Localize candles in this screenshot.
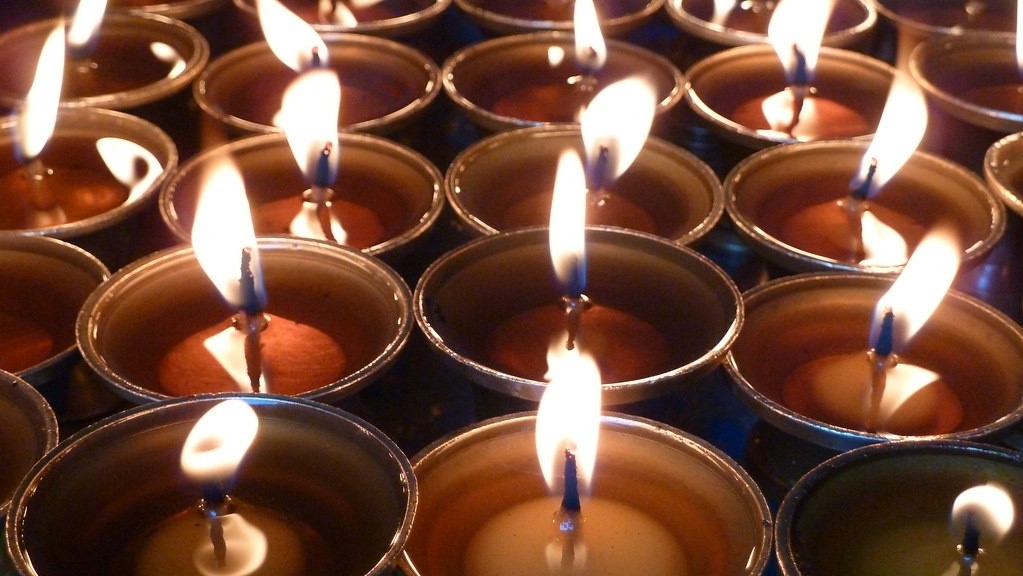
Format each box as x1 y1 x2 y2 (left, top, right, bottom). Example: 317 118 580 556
0 0 1023 576
